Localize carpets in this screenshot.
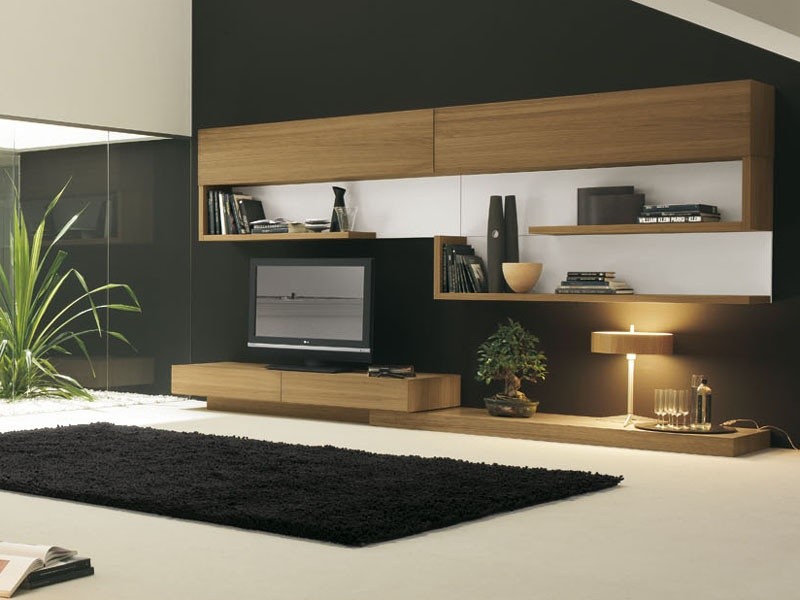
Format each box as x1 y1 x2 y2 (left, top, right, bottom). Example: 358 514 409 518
0 422 626 549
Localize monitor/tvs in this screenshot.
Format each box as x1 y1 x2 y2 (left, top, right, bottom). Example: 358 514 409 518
248 257 374 373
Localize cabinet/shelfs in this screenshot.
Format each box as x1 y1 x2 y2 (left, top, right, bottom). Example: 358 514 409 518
197 78 775 308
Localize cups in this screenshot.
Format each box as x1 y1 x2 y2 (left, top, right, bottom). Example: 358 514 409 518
334 206 358 232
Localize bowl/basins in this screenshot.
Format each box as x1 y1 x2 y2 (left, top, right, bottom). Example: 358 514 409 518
501 262 543 293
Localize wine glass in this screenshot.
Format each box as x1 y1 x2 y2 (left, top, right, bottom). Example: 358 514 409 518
652 374 705 431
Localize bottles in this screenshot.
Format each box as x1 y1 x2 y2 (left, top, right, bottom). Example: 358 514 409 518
330 186 349 233
694 378 713 431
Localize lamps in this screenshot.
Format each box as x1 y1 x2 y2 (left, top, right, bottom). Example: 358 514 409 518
590 326 676 427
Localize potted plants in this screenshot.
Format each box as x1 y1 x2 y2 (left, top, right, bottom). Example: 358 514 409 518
473 319 548 417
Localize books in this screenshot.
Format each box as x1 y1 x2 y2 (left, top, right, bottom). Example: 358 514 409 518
0 542 95 598
555 272 635 294
208 191 267 235
442 243 487 293
638 203 721 222
249 218 290 233
